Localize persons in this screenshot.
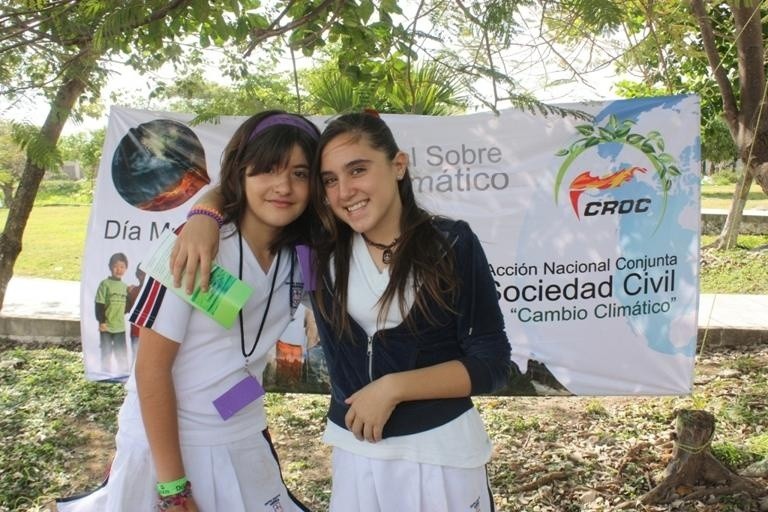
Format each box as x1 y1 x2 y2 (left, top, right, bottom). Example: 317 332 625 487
168 106 512 512
125 261 147 368
274 303 316 392
55 109 322 512
95 252 128 372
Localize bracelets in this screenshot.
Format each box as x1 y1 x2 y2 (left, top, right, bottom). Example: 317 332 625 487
188 203 225 228
154 478 192 511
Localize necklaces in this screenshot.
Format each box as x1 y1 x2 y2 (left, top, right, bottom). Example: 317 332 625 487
360 231 398 264
